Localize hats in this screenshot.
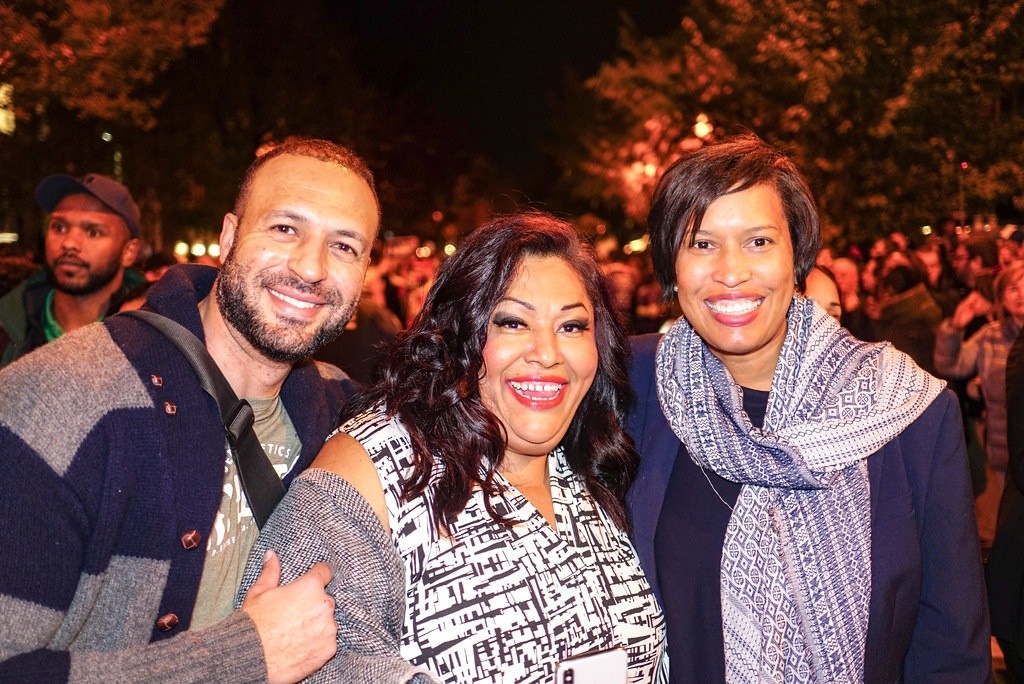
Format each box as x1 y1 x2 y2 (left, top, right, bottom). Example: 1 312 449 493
35 173 141 238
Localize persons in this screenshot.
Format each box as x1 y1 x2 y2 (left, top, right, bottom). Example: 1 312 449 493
0 136 1024 684
232 210 673 684
